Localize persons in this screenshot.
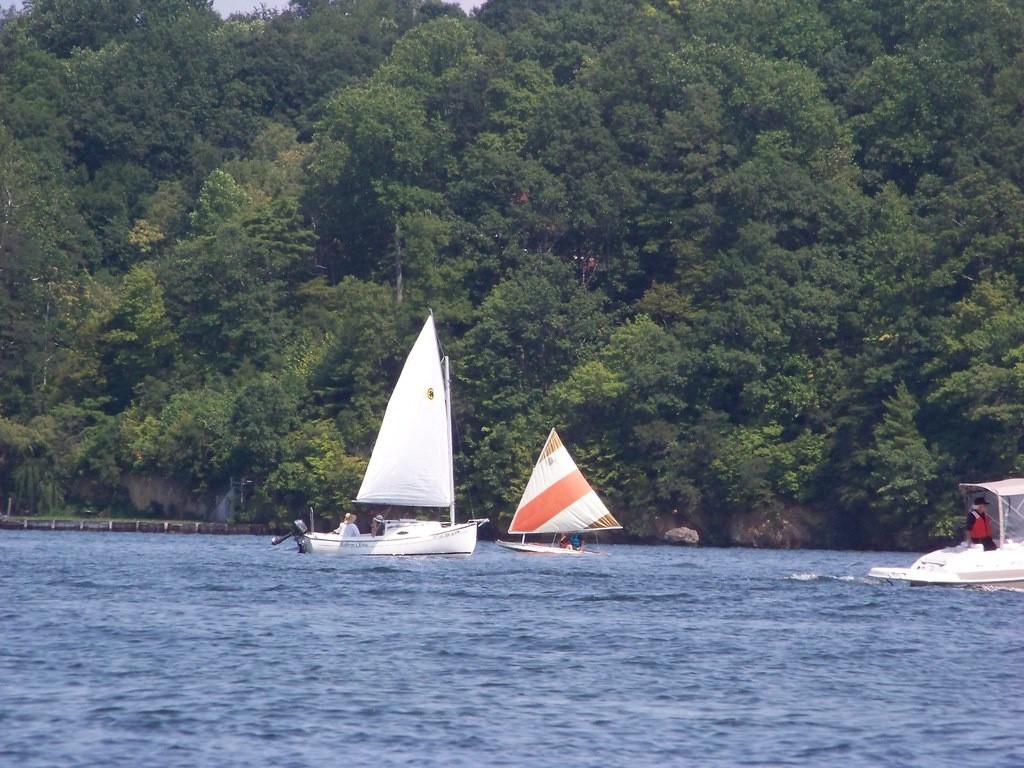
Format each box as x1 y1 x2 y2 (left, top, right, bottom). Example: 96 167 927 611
560 532 585 551
334 513 360 536
374 514 384 535
966 498 997 551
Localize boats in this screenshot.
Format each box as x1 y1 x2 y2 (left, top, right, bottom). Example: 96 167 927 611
867 478 1024 591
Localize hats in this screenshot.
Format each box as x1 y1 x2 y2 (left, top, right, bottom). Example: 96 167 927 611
344 513 357 524
373 515 383 523
974 497 991 505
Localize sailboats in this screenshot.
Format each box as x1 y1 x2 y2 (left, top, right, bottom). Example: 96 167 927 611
269 307 491 557
494 426 623 556
